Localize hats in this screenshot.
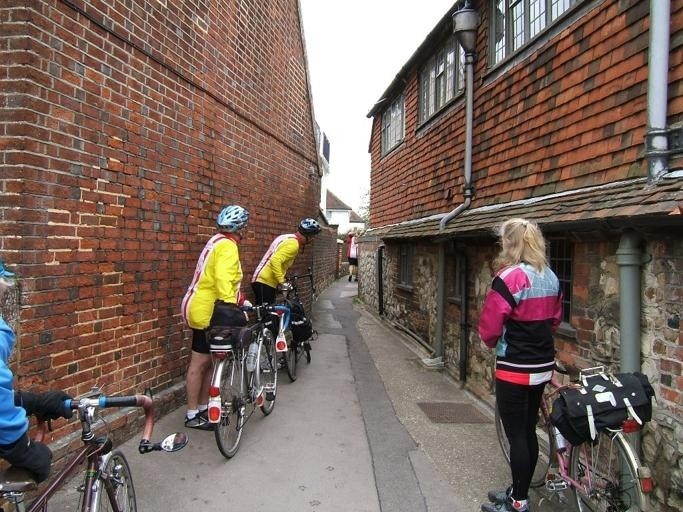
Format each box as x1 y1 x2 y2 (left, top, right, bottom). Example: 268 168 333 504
0 259 15 279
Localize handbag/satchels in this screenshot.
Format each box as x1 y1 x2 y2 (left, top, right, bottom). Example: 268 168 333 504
209 299 246 326
290 315 313 342
548 371 655 446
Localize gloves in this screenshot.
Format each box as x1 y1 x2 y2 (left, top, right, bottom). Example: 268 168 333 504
280 282 293 291
14 388 73 431
0 431 52 483
238 299 252 309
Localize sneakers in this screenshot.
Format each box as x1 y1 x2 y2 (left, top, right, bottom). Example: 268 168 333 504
198 407 231 425
183 412 214 430
487 483 513 505
481 496 530 512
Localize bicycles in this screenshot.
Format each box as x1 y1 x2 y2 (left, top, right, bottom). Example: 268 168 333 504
196 301 279 462
483 312 656 506
0 386 180 506
269 273 320 378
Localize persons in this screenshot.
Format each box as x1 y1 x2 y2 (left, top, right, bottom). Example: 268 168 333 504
0 258 71 484
179 202 251 432
251 217 321 371
172 434 191 451
476 217 566 512
345 230 357 281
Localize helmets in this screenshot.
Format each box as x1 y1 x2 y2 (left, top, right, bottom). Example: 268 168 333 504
215 205 249 234
297 217 323 238
